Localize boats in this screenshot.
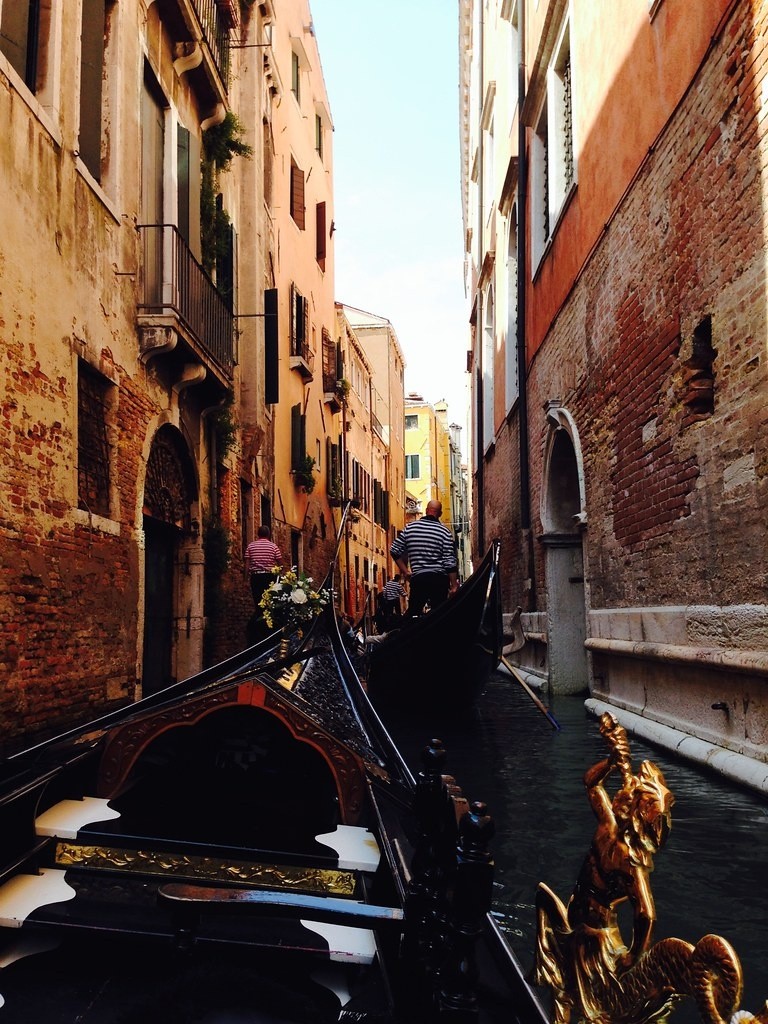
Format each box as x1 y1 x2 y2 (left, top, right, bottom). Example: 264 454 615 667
367 535 505 715
0 500 768 1024
246 575 371 659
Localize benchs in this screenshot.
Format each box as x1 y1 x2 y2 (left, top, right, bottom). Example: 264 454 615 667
0 896 380 964
33 796 381 872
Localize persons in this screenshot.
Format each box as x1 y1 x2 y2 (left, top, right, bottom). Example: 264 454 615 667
383 574 409 618
244 527 282 622
336 610 398 654
390 500 459 619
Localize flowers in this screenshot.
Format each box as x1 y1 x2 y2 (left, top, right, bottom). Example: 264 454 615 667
258 564 330 628
